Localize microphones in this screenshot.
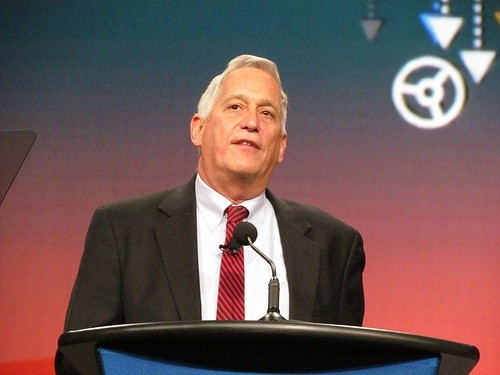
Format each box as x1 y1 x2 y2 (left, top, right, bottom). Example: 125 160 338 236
228 221 288 322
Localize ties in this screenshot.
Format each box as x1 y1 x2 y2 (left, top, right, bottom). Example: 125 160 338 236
215 206 249 320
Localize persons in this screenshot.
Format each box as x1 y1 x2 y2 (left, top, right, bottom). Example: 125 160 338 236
54 53 366 375
